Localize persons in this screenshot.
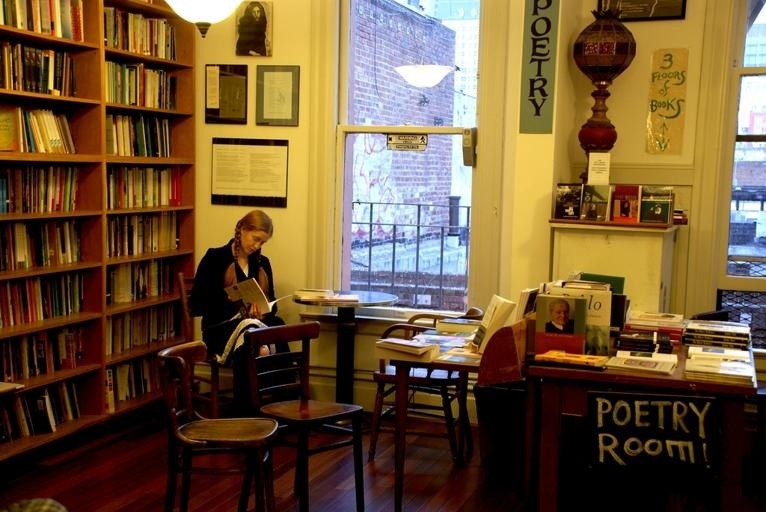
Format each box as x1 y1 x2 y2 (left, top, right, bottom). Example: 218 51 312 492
188 210 301 417
235 1 272 57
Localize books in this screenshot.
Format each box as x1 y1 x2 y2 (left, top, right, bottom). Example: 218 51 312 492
296 284 361 302
552 182 689 227
374 326 483 366
0 352 177 444
0 0 176 60
469 293 517 354
3 42 177 110
0 165 183 215
0 258 181 329
1 104 171 157
0 301 183 393
0 211 179 272
516 270 758 387
225 277 295 321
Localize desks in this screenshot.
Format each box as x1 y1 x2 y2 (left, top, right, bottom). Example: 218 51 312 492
378 323 540 512
509 337 759 512
296 287 403 434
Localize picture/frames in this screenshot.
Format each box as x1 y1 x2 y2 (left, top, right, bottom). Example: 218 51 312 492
597 0 687 21
254 64 299 128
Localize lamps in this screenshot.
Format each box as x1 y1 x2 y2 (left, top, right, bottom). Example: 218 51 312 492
395 20 455 93
571 6 641 191
164 0 243 38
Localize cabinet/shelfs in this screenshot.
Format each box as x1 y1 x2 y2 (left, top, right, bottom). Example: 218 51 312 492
0 0 201 473
549 215 689 317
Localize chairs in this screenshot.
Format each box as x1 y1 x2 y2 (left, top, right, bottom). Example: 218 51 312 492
233 319 374 512
151 334 281 512
369 305 487 474
174 270 281 421
